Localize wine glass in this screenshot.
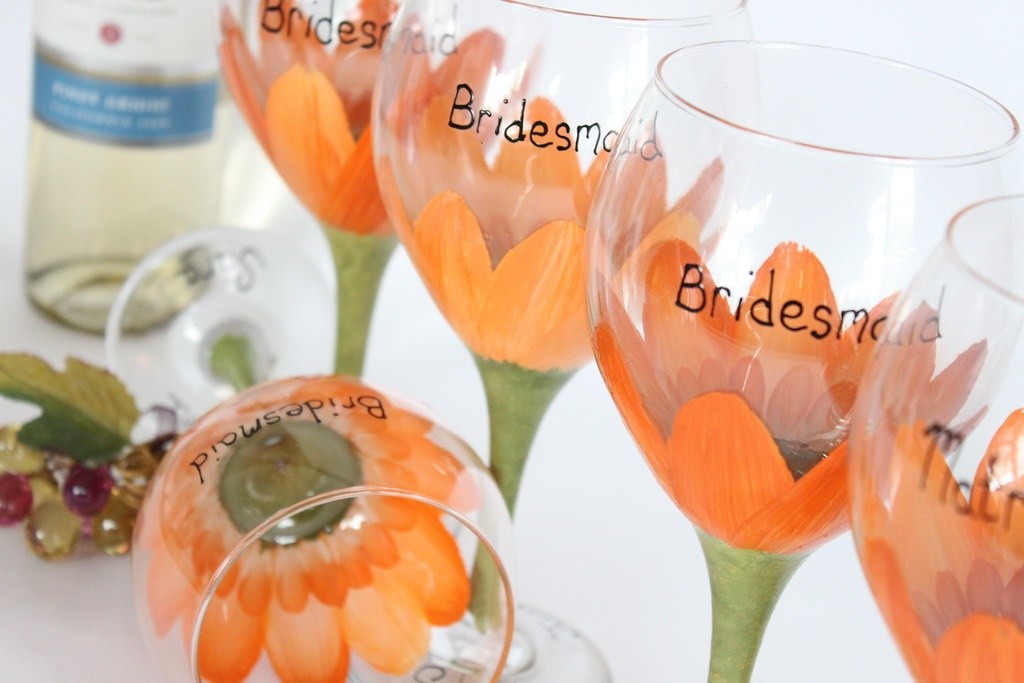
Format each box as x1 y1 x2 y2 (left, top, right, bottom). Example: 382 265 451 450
106 0 1023 683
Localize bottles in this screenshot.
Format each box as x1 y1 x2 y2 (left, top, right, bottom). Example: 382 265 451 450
22 0 230 329
